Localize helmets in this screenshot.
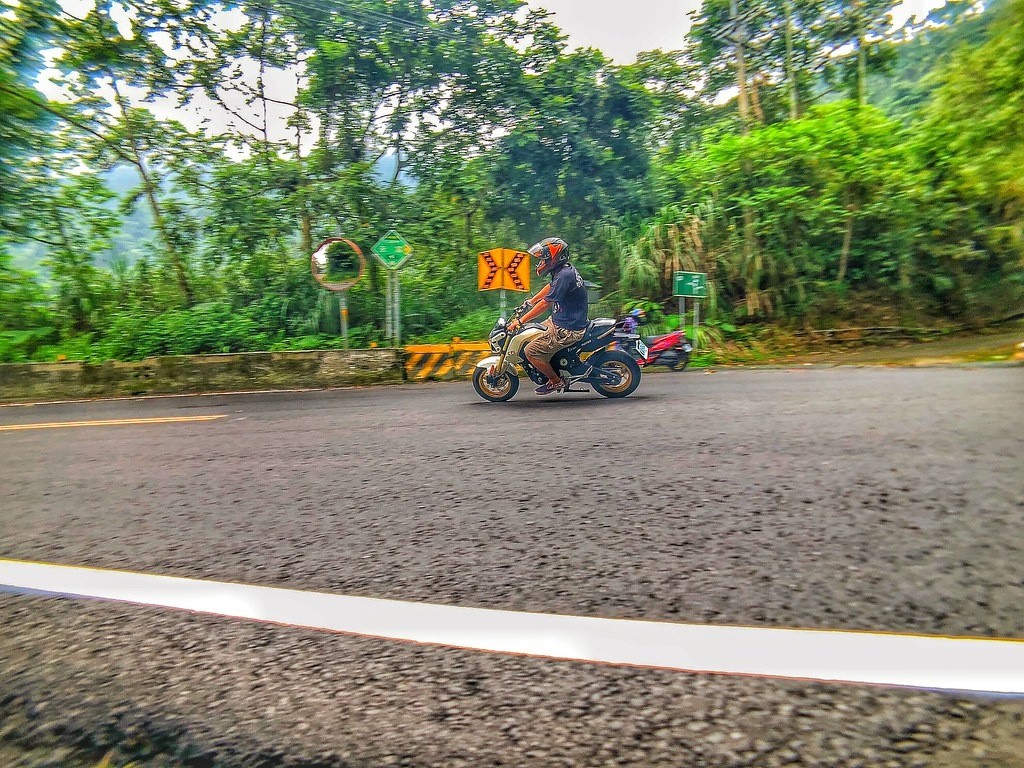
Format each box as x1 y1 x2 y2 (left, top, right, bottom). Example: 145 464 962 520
535 237 569 278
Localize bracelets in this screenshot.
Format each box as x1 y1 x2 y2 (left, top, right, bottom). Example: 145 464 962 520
518 319 523 326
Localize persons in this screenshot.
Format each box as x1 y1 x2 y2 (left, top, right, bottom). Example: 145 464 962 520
508 237 588 395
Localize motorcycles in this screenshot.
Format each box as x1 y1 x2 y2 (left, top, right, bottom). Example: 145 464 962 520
614 310 691 370
472 300 649 403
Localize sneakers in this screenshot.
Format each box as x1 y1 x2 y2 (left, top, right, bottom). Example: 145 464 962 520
534 379 565 395
